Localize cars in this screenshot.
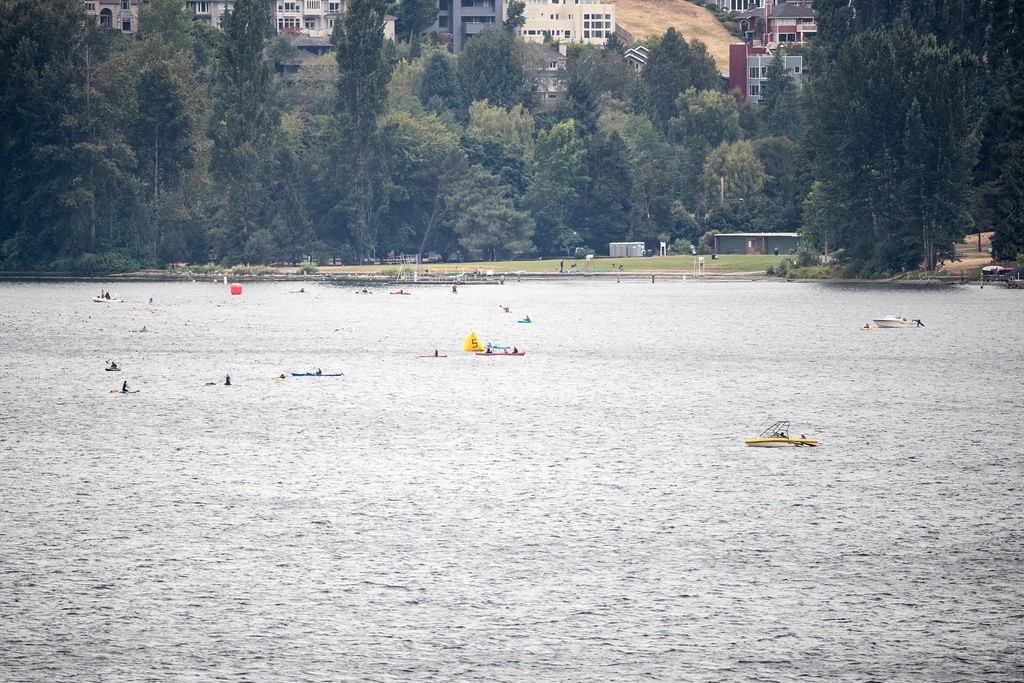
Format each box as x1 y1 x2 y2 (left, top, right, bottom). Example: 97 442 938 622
979 266 1015 281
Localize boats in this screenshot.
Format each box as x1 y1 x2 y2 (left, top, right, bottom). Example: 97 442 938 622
517 319 532 323
105 367 122 371
129 330 159 332
485 343 511 349
420 355 447 357
745 421 818 448
109 389 141 393
390 292 412 295
476 351 526 355
91 297 122 302
290 291 310 293
356 292 372 294
873 315 918 328
203 382 232 387
291 372 343 376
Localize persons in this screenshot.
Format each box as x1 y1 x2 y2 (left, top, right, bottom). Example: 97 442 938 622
864 324 869 328
525 316 529 320
315 367 322 374
110 361 117 368
486 347 493 353
452 284 457 292
279 374 286 378
435 349 438 356
101 290 110 299
570 262 577 273
122 380 130 392
512 347 518 354
559 260 564 273
